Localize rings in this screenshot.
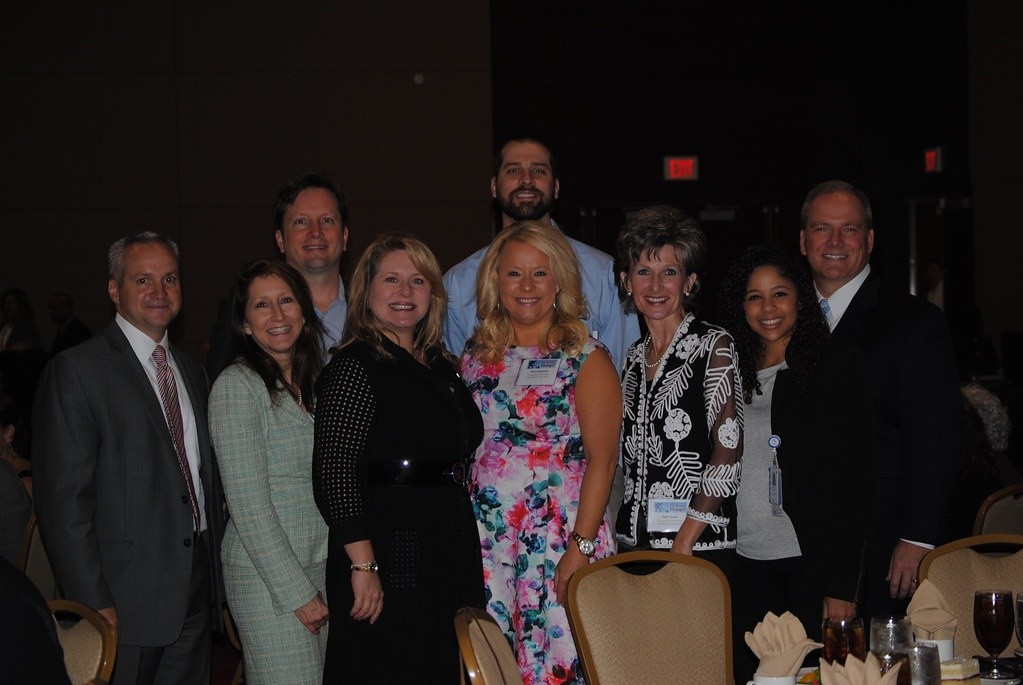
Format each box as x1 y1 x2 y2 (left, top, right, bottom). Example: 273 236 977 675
912 578 918 582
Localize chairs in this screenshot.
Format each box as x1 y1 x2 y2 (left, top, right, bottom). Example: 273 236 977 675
453 606 523 685
563 550 734 685
971 483 1023 557
918 533 1023 659
47 599 118 685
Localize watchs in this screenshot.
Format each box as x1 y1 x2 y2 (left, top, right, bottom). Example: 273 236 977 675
571 532 597 558
350 561 379 572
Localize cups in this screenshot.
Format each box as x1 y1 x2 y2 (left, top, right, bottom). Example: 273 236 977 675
870 618 912 685
1016 592 1023 648
823 617 865 666
911 642 941 685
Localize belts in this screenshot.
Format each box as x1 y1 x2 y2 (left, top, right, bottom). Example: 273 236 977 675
365 456 474 489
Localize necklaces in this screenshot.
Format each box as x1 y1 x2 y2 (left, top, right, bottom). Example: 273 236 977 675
298 389 302 406
644 334 662 368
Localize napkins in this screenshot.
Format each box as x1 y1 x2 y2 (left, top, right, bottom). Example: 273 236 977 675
744 611 824 678
818 651 902 685
904 578 959 640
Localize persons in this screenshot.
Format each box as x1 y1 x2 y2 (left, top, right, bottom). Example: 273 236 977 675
0 295 71 685
709 252 824 685
273 176 361 363
33 233 224 685
206 262 329 685
311 235 485 685
921 264 954 308
959 362 1011 453
438 135 641 385
614 211 745 577
458 222 624 685
787 179 951 662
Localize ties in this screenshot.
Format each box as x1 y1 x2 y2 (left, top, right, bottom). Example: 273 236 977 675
819 299 830 317
151 345 201 538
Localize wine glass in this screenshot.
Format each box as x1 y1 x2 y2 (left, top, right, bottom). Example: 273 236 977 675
974 590 1014 679
